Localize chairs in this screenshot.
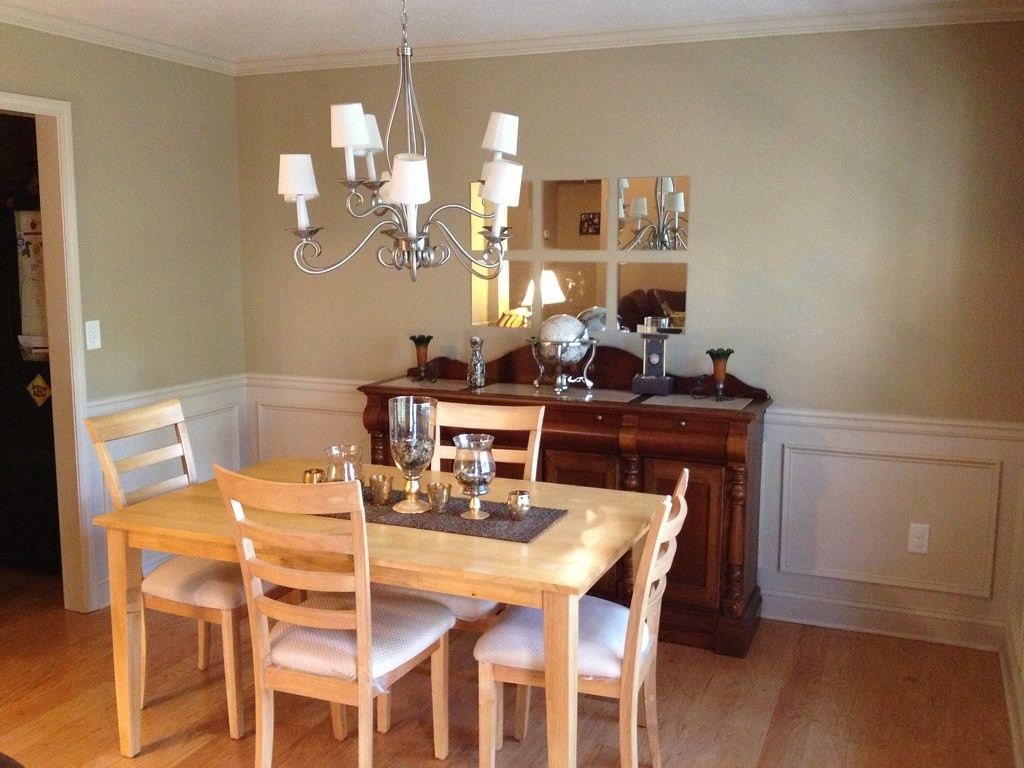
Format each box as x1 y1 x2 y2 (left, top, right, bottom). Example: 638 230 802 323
474 466 690 766
358 399 549 751
213 460 456 768
87 399 321 740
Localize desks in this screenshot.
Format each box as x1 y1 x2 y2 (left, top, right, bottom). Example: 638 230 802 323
92 460 677 768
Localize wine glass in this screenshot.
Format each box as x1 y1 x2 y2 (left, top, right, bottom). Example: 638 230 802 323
452 434 496 520
387 395 438 515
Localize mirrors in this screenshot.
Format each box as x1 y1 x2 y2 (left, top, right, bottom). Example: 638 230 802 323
462 175 691 337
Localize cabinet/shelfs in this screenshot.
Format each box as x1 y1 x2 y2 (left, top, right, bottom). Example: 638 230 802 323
357 343 772 657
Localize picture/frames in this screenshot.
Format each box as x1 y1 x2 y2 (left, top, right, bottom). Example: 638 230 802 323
579 212 601 233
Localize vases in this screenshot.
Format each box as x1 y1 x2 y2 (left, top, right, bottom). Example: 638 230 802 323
706 349 736 401
408 334 438 382
466 336 487 387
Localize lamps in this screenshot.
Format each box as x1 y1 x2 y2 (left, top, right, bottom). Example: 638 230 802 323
618 177 688 251
272 2 524 282
521 267 565 325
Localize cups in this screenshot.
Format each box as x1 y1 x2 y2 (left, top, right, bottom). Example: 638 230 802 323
303 469 328 483
427 482 452 514
507 490 531 521
369 475 393 505
323 445 366 492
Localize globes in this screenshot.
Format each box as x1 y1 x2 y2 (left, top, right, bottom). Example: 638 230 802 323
526 313 598 395
